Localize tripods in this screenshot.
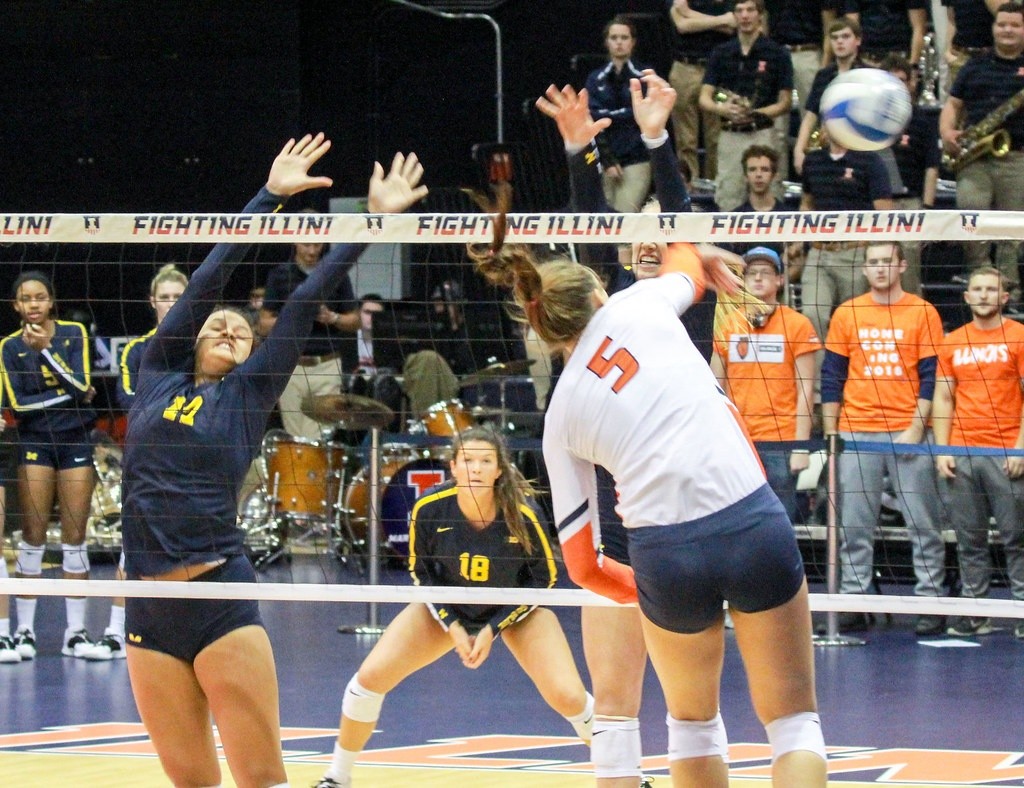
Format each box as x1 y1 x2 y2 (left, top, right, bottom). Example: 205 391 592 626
258 430 364 566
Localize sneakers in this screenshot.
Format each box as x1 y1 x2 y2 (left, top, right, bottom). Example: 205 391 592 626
311 771 351 788
90 626 128 660
914 615 948 635
13 628 37 660
0 634 21 663
816 612 868 632
946 617 993 636
61 628 95 657
1014 620 1024 638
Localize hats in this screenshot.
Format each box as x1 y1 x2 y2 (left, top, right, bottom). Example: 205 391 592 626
742 246 781 275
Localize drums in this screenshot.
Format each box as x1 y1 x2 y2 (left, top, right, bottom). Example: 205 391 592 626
345 442 455 545
269 441 348 523
421 398 474 441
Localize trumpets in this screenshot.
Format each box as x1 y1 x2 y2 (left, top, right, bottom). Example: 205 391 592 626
917 33 944 111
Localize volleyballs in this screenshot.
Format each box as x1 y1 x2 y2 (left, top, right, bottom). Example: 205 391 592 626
821 68 915 151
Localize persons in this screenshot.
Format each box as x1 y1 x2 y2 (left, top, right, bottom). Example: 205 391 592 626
120 129 429 788
0 0 1024 788
709 244 821 630
312 425 654 788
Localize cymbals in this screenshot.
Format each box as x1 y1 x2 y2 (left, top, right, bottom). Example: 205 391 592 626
456 359 540 387
299 392 394 435
463 404 511 418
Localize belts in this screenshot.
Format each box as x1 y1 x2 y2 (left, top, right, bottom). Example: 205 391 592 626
294 350 340 367
811 241 869 251
721 120 773 132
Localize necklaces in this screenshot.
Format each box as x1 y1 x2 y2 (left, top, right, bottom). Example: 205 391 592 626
748 305 773 330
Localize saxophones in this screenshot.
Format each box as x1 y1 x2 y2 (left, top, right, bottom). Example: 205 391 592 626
940 83 1024 168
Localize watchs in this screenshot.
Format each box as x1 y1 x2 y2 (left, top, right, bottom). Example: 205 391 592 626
331 313 340 325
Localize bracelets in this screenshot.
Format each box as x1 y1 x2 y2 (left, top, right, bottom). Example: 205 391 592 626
923 204 935 211
789 445 810 455
